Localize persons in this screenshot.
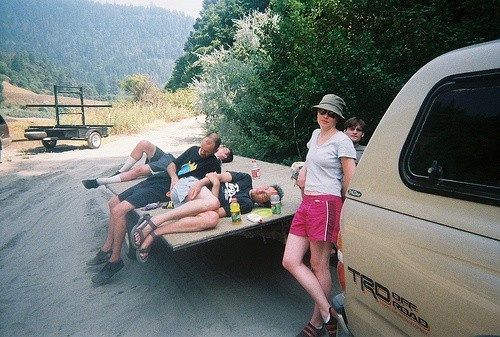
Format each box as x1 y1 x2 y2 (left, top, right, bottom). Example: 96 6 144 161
344 115 367 165
86 132 221 284
82 140 233 188
283 94 357 337
130 171 284 263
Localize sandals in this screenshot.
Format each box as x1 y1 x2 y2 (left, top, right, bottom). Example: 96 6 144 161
130 213 157 249
136 231 158 263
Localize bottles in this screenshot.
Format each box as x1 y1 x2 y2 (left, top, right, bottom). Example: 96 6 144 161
229 198 242 225
270 191 281 215
251 160 260 179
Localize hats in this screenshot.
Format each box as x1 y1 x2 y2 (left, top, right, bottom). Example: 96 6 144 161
312 94 346 120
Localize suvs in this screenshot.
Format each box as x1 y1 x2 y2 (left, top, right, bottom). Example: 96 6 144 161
0 113 13 154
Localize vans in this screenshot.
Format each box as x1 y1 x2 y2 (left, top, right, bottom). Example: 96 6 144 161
339 37 500 337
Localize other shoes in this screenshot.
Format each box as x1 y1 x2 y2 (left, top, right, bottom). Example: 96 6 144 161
295 322 324 337
322 306 339 337
82 179 100 189
111 171 122 177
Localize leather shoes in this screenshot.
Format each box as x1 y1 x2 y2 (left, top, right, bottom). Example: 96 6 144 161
91 258 125 286
86 248 113 265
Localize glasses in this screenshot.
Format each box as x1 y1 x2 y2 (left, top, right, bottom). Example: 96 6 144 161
317 108 336 118
